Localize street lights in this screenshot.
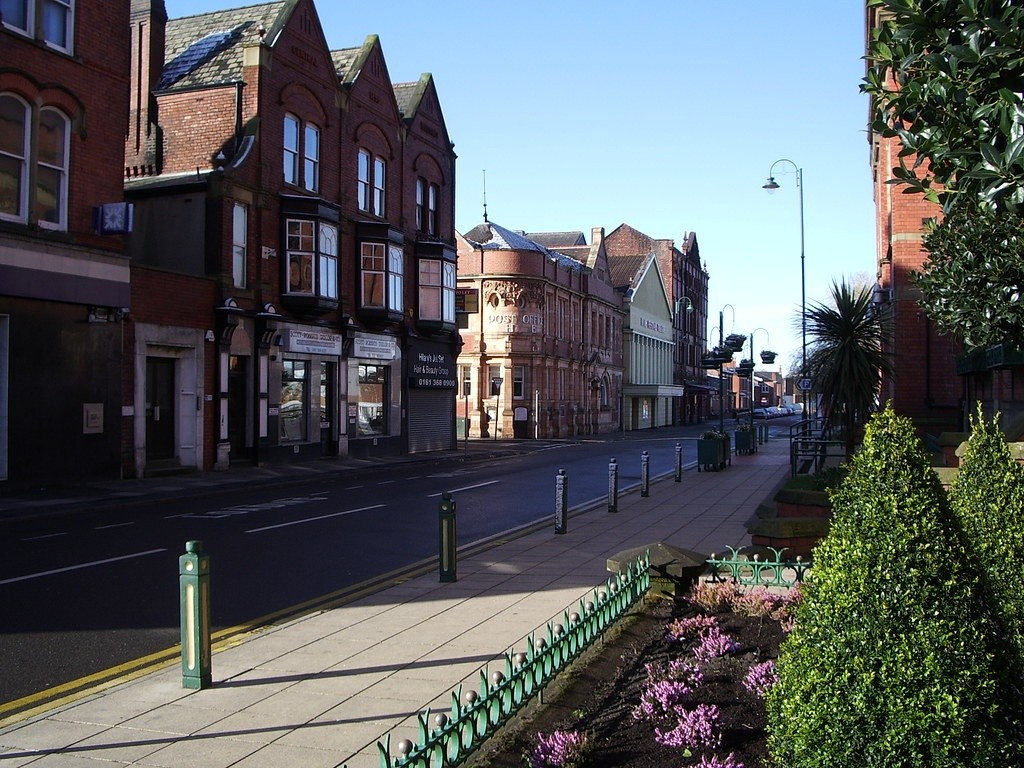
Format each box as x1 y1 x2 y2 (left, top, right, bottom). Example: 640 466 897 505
675 295 693 428
761 158 811 450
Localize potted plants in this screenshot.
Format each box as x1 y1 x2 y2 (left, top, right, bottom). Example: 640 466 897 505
759 350 779 363
734 425 758 456
701 333 755 378
697 429 731 473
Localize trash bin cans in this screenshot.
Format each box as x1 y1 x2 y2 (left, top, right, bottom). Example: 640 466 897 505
456 418 470 437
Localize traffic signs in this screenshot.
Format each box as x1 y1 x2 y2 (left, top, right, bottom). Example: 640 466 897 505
796 378 812 390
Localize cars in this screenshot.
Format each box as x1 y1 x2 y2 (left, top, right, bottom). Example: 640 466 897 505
750 403 804 420
